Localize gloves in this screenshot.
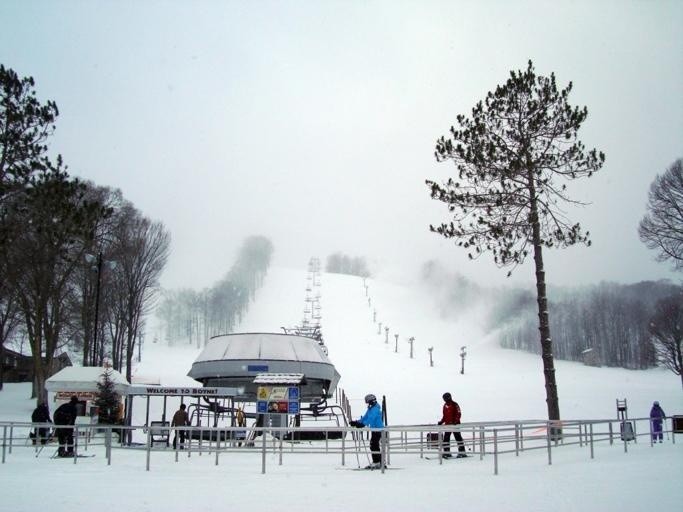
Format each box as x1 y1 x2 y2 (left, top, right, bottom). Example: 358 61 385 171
349 421 364 428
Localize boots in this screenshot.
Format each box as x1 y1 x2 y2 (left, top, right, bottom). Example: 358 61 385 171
365 463 387 469
58 451 74 457
441 454 468 457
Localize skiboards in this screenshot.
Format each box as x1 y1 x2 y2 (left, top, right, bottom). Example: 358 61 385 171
425 456 474 460
50 453 96 459
351 468 402 471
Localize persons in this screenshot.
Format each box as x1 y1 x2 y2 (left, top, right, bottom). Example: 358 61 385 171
436 392 468 458
350 393 383 469
31 399 53 445
53 394 79 457
649 401 666 444
171 403 190 449
110 392 125 443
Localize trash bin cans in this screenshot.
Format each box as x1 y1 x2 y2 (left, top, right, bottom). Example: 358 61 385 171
427 433 444 449
673 415 683 434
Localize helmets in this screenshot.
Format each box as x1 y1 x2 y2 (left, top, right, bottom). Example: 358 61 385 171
365 394 376 403
